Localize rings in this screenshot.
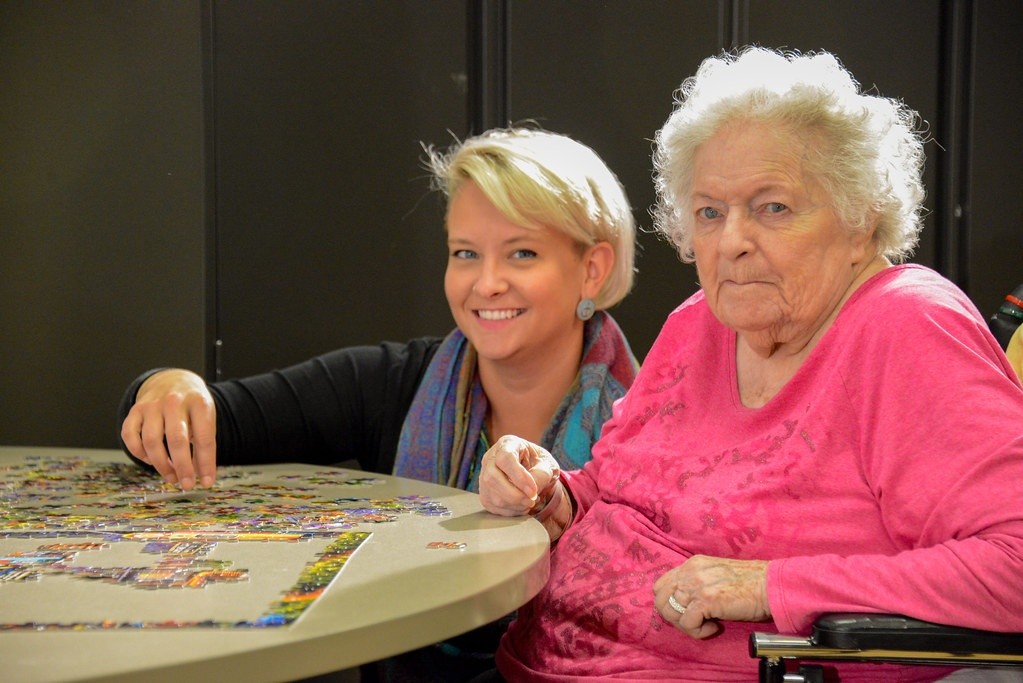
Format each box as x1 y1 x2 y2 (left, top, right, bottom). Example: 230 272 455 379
668 595 686 614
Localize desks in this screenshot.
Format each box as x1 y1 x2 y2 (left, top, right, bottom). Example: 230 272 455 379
0 446 550 683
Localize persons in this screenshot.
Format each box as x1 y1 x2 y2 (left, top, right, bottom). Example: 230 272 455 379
480 49 1023 683
117 120 639 683
